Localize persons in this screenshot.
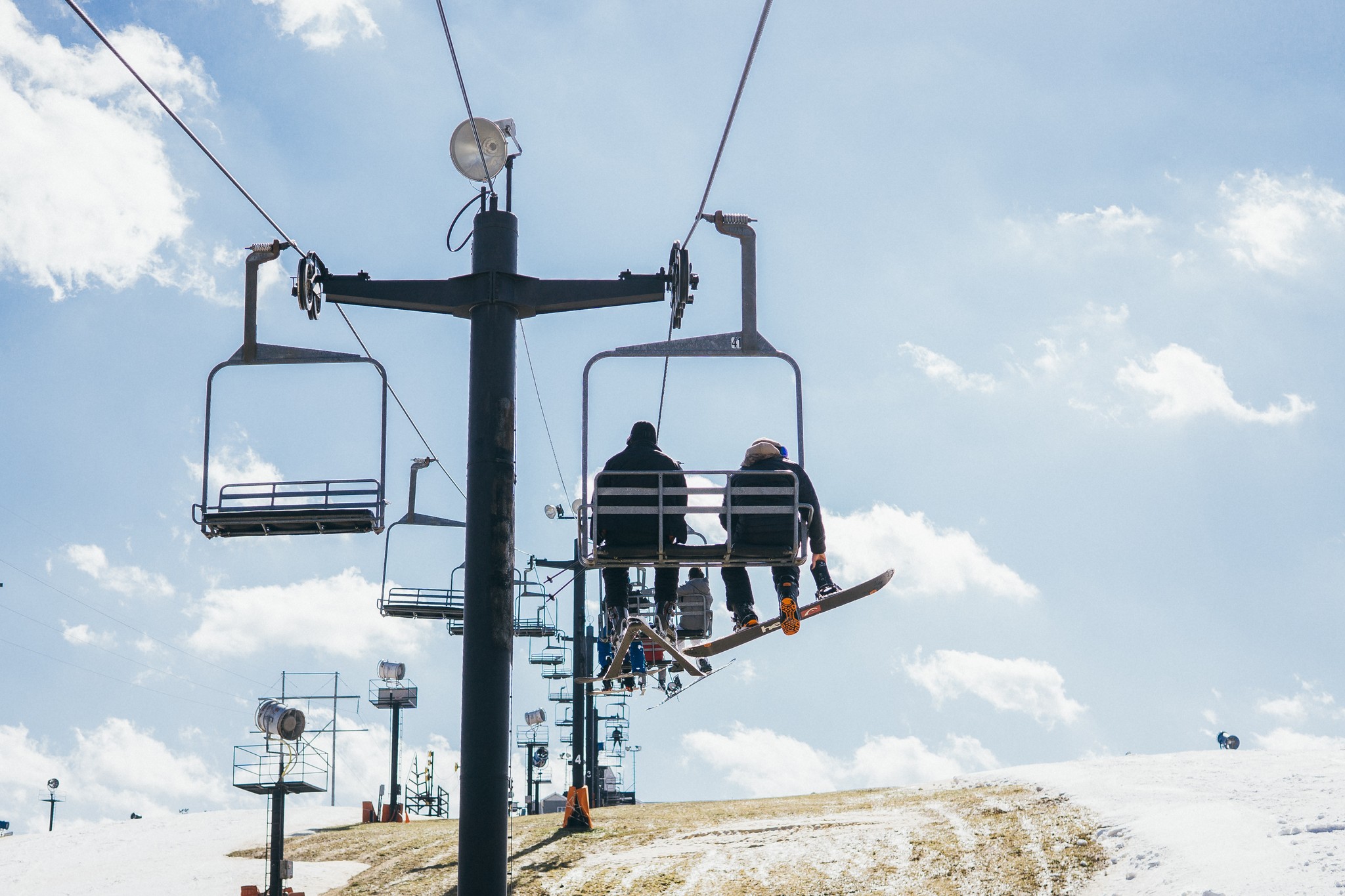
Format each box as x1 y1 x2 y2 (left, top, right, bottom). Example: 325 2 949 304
602 652 633 692
669 567 714 675
597 583 650 676
612 727 622 746
637 621 666 690
719 438 827 635
590 420 687 640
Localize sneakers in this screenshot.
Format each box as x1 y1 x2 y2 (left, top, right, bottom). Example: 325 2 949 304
601 685 613 691
640 683 645 691
633 670 648 676
598 656 612 677
776 574 801 635
653 600 677 641
626 686 633 692
658 680 666 690
608 606 629 648
700 660 712 674
668 663 684 672
731 602 758 633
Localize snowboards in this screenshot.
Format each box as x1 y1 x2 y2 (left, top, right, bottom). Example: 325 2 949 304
573 665 669 684
645 658 737 711
681 567 896 658
586 683 652 696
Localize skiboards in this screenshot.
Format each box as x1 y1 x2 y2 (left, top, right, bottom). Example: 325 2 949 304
602 617 707 681
651 687 669 694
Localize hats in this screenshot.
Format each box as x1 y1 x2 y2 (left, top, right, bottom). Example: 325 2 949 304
626 421 656 445
752 437 780 447
